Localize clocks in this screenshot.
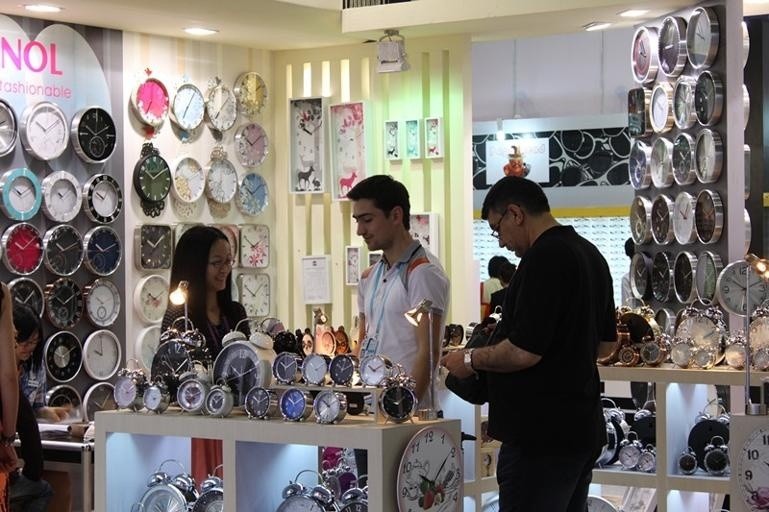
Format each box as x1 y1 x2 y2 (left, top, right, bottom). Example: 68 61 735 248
0 98 123 418
130 67 270 324
114 316 463 512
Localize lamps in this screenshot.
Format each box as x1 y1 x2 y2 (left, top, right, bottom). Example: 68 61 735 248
405 299 438 420
312 307 328 354
496 117 505 141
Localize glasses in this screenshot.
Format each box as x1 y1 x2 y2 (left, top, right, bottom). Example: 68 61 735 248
491 209 507 237
209 258 234 268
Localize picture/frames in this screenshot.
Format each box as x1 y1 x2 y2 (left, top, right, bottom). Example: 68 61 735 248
424 117 444 159
299 255 332 304
405 120 420 158
383 120 400 159
367 252 382 266
410 214 438 256
344 246 359 284
288 97 325 194
328 101 367 202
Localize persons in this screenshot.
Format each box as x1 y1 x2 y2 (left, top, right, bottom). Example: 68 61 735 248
440 175 617 512
159 225 251 370
481 256 516 325
0 283 53 511
503 146 530 177
621 238 634 310
346 174 450 485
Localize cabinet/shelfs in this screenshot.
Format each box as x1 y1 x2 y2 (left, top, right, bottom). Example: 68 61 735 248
463 369 768 511
94 408 461 512
14 424 93 511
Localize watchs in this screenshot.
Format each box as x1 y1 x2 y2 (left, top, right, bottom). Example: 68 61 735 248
463 348 478 374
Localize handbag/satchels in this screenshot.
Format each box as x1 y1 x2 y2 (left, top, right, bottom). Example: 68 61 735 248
445 318 497 405
